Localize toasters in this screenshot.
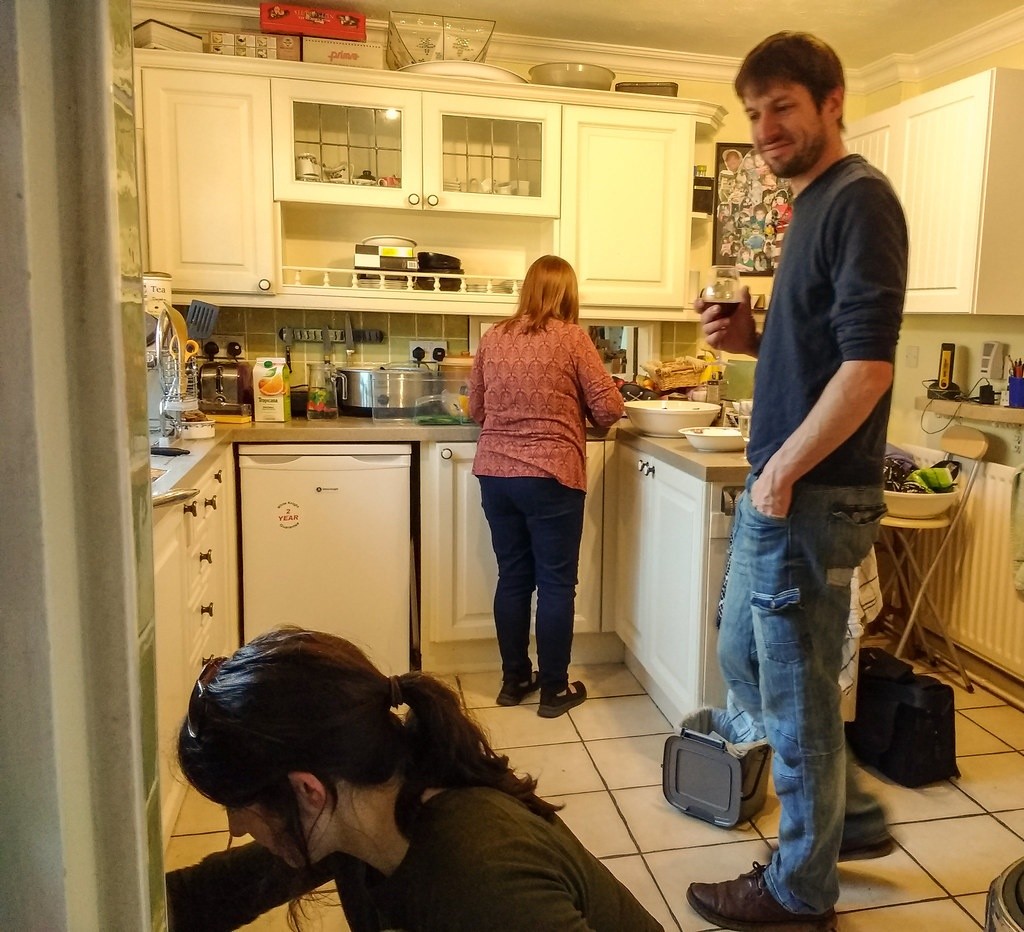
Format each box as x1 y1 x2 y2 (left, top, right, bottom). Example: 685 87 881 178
198 361 248 416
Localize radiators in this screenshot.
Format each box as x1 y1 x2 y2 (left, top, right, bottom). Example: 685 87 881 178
886 442 1024 682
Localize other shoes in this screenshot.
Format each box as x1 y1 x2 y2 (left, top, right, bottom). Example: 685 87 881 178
537 681 586 717
496 670 542 705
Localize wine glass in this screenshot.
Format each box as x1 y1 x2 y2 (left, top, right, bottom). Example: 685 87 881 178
738 400 754 461
703 264 743 367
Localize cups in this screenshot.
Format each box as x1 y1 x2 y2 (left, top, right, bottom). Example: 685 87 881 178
495 182 512 195
1008 377 1024 408
297 152 354 183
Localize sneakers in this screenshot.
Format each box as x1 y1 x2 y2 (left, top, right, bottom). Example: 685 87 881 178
836 838 893 863
686 860 839 932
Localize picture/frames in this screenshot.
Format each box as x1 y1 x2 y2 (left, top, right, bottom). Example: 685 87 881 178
712 142 795 277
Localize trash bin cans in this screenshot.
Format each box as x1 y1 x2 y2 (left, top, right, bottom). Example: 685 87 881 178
662 706 773 828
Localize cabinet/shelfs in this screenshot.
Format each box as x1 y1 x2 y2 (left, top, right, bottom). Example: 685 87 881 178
615 443 858 736
840 68 1024 315
152 444 239 855
270 60 561 218
131 48 282 309
426 440 626 674
542 87 730 323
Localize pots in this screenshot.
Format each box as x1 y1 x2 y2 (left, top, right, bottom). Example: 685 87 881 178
338 367 432 419
438 351 475 420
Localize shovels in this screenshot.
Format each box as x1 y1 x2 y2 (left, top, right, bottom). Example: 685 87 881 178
186 299 220 353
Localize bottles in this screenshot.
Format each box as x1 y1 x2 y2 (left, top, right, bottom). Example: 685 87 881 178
697 165 707 176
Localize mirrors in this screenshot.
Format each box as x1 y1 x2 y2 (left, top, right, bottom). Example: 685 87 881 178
589 326 638 377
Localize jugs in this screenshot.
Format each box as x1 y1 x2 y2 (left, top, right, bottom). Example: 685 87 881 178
306 362 347 421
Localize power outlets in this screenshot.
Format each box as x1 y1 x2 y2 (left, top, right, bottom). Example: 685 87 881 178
409 341 447 362
202 334 245 359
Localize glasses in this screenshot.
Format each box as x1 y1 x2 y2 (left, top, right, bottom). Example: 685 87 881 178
186 656 318 761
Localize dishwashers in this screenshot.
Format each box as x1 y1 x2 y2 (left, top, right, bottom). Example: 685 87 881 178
236 443 410 679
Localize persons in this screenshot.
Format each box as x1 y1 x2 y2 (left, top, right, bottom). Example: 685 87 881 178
468 254 624 718
166 628 665 932
686 30 910 932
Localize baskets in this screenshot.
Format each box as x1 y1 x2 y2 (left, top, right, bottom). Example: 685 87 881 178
650 359 708 391
160 357 200 413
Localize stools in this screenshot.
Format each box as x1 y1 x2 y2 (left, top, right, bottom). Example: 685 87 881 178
879 425 989 694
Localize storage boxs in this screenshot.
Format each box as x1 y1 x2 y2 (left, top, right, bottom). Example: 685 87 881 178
130 0 383 69
355 244 418 271
707 379 739 428
615 82 679 96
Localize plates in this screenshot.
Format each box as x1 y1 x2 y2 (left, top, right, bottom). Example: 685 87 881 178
357 278 416 290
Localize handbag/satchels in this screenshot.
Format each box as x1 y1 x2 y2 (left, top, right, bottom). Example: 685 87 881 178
844 647 961 788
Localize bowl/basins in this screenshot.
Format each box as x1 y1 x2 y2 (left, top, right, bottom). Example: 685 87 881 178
361 235 417 248
417 252 464 292
677 426 742 451
622 400 721 438
883 490 958 520
528 63 616 91
180 420 216 440
